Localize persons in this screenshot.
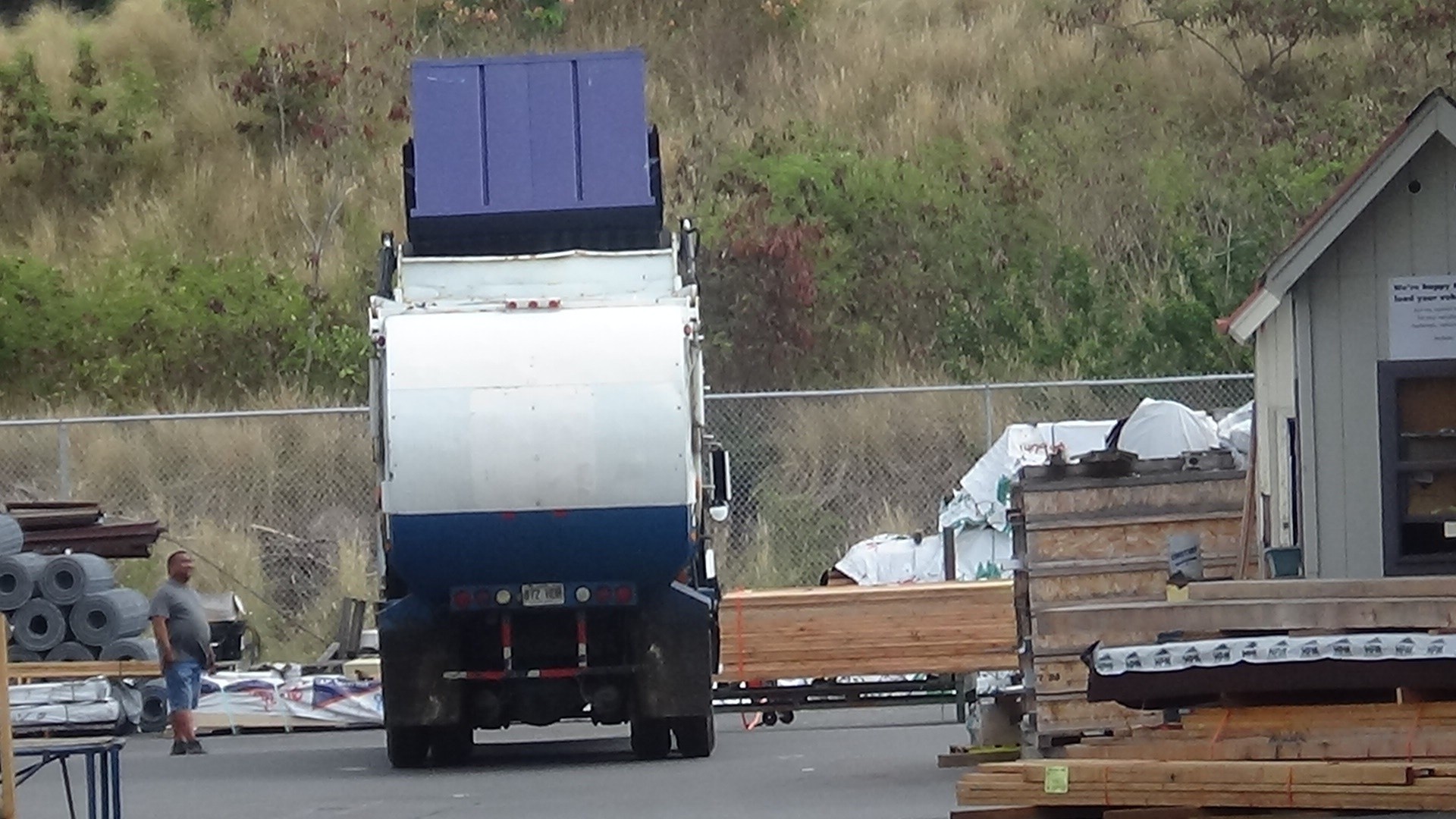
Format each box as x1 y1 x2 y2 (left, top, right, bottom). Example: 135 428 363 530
148 549 213 754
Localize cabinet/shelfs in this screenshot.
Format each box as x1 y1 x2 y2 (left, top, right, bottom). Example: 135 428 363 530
1376 358 1456 577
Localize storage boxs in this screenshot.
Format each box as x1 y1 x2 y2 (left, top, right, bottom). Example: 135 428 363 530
1266 544 1301 578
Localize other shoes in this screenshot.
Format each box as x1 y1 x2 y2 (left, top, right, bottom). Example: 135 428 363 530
187 740 203 753
173 741 189 754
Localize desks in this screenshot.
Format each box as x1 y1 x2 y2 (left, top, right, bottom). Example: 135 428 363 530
12 736 124 819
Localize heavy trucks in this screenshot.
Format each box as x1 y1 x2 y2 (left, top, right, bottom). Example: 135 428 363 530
363 51 730 768
